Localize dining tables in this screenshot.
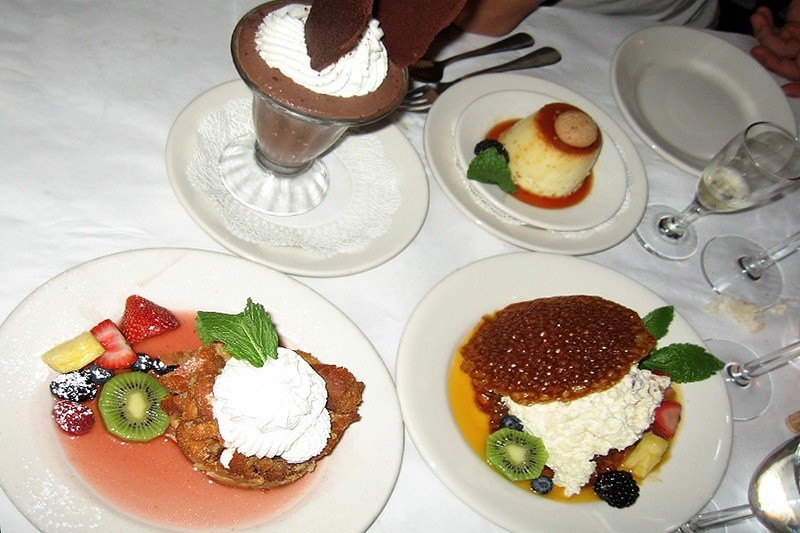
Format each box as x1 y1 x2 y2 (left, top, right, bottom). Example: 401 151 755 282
0 0 800 533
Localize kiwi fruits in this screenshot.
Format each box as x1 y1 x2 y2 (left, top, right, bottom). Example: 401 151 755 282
487 428 548 481
97 370 171 442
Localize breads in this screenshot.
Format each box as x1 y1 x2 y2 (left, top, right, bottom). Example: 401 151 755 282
160 343 364 489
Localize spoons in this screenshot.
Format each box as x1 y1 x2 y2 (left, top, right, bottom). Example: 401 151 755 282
406 32 535 83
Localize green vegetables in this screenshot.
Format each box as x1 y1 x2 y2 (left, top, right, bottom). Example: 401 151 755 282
194 297 279 367
638 303 727 383
467 148 520 193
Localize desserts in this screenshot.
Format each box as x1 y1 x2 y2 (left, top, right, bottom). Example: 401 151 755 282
496 102 602 198
233 0 469 124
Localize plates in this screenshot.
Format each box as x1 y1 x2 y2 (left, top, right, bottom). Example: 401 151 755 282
163 78 431 277
0 248 405 533
392 251 735 532
611 25 798 180
421 71 648 257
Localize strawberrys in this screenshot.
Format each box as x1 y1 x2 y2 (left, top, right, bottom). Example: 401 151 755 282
651 400 681 438
54 401 96 436
119 295 180 342
87 319 137 369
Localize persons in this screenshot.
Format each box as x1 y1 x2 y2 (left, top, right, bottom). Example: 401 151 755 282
453 0 800 97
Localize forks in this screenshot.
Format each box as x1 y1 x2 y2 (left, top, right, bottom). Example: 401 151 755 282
396 46 562 112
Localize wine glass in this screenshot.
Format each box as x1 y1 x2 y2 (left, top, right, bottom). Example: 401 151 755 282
669 432 800 533
218 0 411 216
633 121 800 263
705 339 800 423
700 230 800 314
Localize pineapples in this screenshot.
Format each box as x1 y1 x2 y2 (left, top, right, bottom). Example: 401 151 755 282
620 433 668 478
41 332 106 373
